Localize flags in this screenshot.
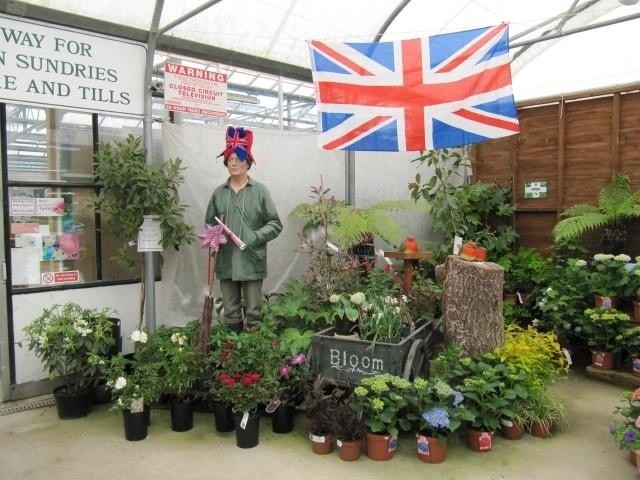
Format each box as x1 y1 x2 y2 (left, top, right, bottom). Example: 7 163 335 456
308 24 521 151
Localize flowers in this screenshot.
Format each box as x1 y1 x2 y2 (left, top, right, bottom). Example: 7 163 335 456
329 291 411 355
104 319 316 416
13 303 120 391
353 345 528 447
497 247 640 360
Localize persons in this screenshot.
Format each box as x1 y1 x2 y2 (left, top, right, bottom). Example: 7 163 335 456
205 126 283 331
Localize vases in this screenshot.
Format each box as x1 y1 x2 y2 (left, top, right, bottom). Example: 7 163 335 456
416 432 449 464
632 359 640 378
169 395 193 431
233 411 259 448
591 350 613 369
271 401 294 433
365 431 397 460
121 409 147 441
210 399 235 432
52 384 89 419
87 381 112 404
144 403 150 427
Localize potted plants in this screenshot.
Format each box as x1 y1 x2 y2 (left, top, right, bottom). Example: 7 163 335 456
307 372 364 461
552 168 640 254
526 382 570 438
